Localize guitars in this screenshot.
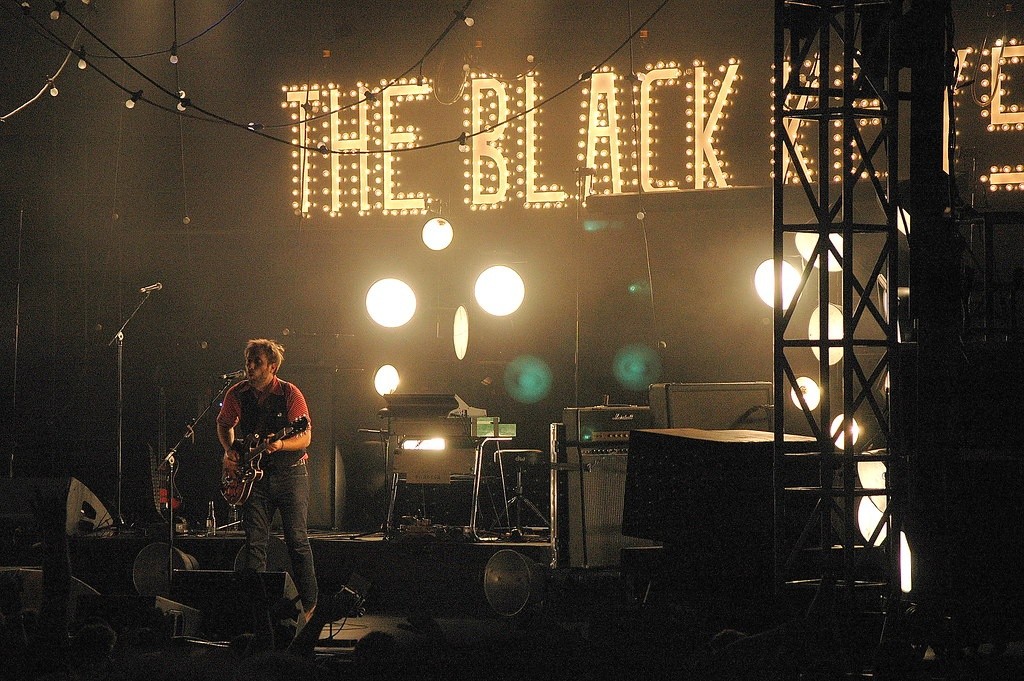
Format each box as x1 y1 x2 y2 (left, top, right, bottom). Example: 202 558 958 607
221 412 309 503
145 381 190 523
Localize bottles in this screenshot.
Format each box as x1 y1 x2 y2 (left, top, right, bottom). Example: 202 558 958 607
206 500 216 537
228 504 238 531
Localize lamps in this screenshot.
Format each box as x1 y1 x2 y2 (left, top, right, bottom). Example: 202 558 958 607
133 543 198 597
434 43 468 106
421 211 454 252
484 549 545 617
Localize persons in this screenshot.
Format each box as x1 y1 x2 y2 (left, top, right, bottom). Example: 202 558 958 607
216 339 318 612
0 486 746 681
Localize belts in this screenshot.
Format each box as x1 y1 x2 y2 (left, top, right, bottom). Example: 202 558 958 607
262 458 306 467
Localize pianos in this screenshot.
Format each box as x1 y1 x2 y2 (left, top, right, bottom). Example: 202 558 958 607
371 393 487 415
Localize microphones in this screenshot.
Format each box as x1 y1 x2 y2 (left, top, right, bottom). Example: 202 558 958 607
219 370 246 380
140 283 163 293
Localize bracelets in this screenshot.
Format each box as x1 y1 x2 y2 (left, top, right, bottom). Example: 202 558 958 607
280 440 284 449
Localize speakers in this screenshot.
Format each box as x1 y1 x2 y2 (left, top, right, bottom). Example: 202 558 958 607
0 474 115 538
66 594 205 650
333 361 449 536
244 366 334 527
0 564 99 644
168 569 306 646
648 382 777 434
562 443 651 569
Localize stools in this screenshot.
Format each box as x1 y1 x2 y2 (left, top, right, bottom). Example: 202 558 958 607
488 449 549 535
